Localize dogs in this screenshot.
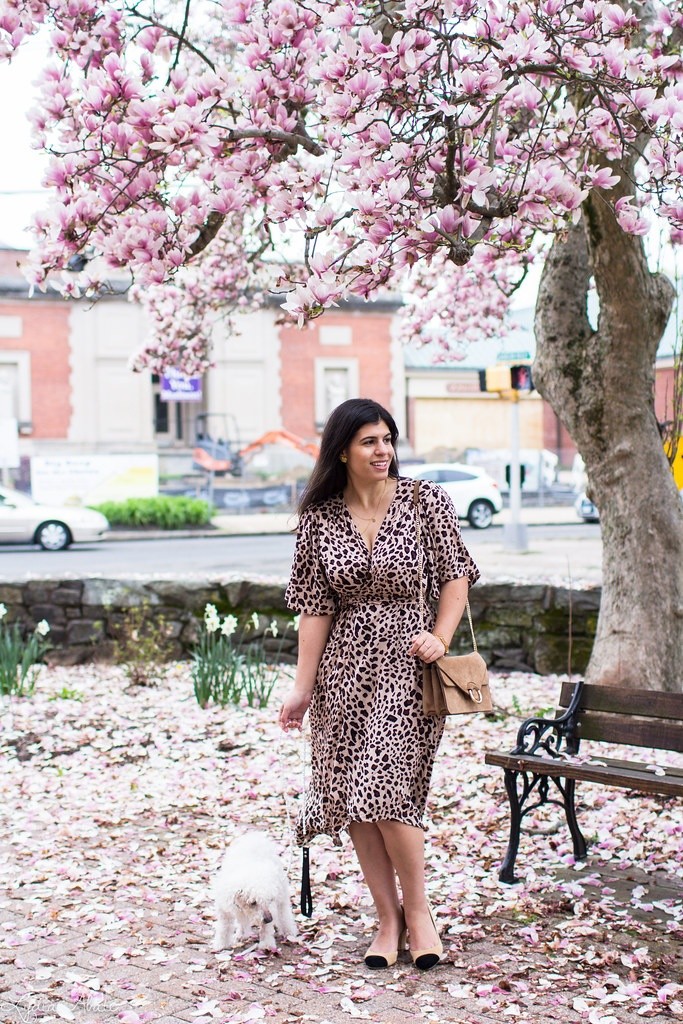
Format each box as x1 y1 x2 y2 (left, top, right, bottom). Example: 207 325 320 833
210 831 299 950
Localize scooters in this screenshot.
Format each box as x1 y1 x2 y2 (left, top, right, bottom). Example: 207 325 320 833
193 414 245 477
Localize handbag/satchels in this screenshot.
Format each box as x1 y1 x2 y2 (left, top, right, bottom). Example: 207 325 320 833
424 651 495 719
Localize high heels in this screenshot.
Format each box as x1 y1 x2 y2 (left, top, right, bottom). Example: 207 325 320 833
408 902 443 969
364 905 406 969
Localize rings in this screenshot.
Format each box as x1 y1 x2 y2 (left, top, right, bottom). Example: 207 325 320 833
424 643 429 649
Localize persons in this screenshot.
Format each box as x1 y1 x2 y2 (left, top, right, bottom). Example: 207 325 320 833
277 398 482 970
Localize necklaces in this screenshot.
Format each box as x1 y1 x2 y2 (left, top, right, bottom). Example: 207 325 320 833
346 479 387 522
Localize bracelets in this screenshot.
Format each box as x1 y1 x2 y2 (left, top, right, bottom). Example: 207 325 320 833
432 633 449 655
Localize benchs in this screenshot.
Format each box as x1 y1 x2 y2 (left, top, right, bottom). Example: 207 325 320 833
484 682 683 884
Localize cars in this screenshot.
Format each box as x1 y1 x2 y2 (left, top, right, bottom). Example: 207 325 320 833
400 466 501 528
0 485 109 551
575 487 600 520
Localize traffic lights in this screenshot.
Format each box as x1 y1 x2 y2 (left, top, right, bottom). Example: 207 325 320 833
486 363 536 391
